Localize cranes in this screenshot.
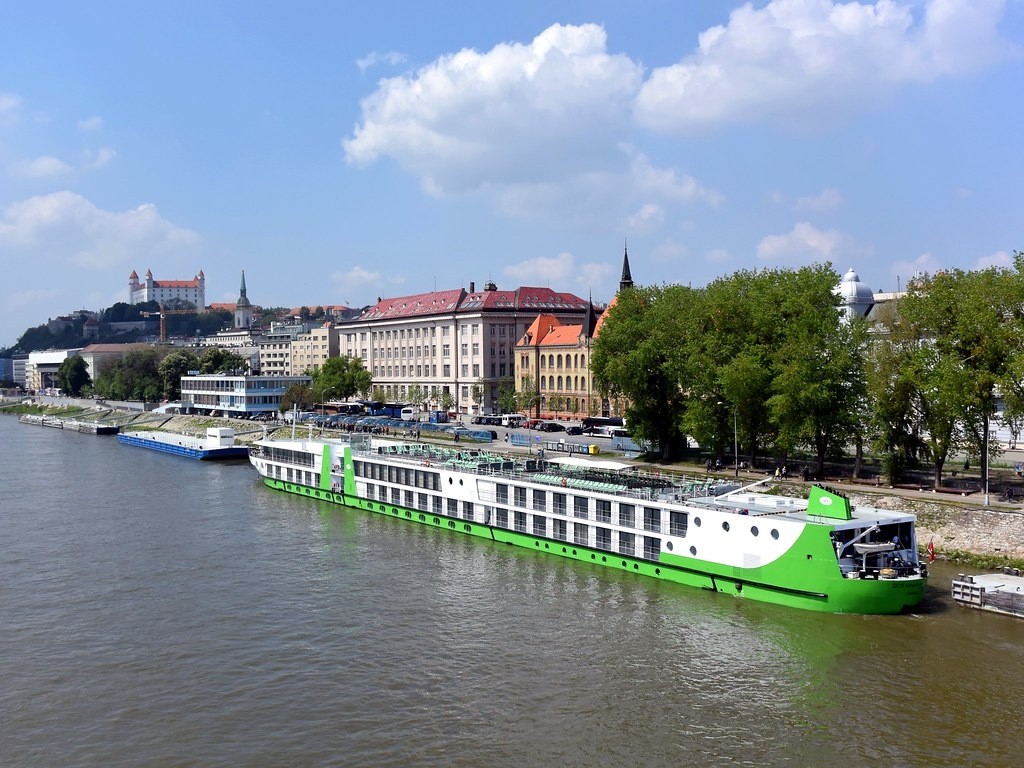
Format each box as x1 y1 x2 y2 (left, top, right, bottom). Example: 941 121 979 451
140 301 227 341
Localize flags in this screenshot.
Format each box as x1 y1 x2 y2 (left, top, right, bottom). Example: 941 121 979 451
927 539 935 565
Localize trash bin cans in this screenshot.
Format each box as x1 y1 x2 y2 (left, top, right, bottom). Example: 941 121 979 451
582 444 588 454
542 441 583 453
589 445 598 454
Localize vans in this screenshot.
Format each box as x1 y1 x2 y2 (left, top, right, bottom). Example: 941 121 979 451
566 427 582 435
530 419 543 430
543 423 565 432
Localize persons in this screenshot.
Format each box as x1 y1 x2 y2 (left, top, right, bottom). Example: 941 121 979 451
568 446 572 457
963 460 970 470
773 466 789 481
1004 487 1014 504
314 419 407 438
453 432 459 444
799 465 810 483
705 458 722 473
504 433 509 443
259 416 294 426
892 534 900 551
409 427 421 440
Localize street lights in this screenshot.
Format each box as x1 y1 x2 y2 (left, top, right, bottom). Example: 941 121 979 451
321 387 335 418
528 394 545 453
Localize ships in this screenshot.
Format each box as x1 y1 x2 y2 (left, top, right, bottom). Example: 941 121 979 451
248 430 926 616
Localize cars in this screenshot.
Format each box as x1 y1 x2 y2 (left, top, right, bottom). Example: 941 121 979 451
535 423 545 430
315 414 470 436
232 413 276 421
523 422 529 428
471 414 502 426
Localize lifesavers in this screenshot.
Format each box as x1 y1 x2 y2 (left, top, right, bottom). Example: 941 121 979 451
561 479 567 487
426 459 430 467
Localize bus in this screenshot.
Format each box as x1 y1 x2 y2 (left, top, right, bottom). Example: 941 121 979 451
314 404 346 418
401 407 419 422
363 401 383 416
612 427 659 453
384 403 412 417
328 401 360 416
502 414 526 427
581 416 626 438
